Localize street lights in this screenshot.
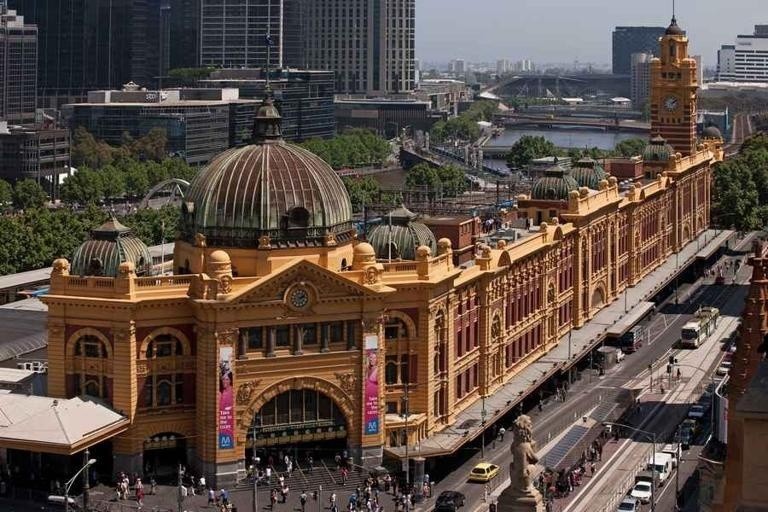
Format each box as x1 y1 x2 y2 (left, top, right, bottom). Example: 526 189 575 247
385 377 450 483
603 419 656 512
252 456 262 512
317 483 322 511
64 458 98 511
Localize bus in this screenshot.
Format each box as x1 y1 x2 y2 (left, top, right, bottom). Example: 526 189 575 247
680 306 720 349
617 326 645 355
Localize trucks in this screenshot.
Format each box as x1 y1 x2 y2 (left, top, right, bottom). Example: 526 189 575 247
646 452 674 486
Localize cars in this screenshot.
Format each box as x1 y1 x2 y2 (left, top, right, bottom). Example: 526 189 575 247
660 441 684 466
46 494 80 512
730 342 737 353
673 383 718 449
436 489 466 512
616 498 642 512
469 463 501 482
631 479 652 504
716 361 730 376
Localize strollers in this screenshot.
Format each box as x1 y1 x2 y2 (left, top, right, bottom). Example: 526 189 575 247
191 476 206 496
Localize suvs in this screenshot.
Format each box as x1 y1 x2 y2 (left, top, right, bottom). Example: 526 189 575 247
634 470 659 493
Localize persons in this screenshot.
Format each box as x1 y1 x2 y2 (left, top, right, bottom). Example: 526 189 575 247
700 258 742 277
113 463 238 512
538 380 568 412
248 448 432 512
538 427 620 501
481 214 504 234
499 425 506 442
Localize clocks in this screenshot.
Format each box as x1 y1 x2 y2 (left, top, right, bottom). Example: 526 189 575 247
284 283 315 313
